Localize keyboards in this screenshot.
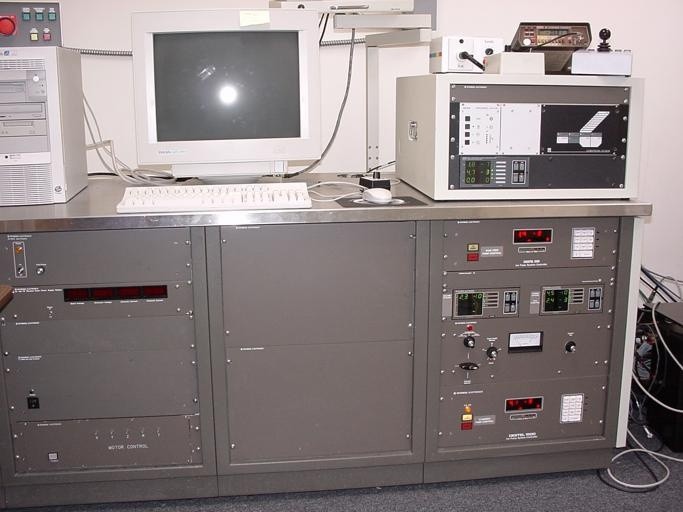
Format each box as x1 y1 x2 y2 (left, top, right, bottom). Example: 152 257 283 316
116 183 312 215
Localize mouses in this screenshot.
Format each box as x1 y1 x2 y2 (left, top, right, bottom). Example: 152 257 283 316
362 187 392 204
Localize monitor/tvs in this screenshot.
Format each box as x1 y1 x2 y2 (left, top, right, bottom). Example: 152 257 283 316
131 9 322 182
